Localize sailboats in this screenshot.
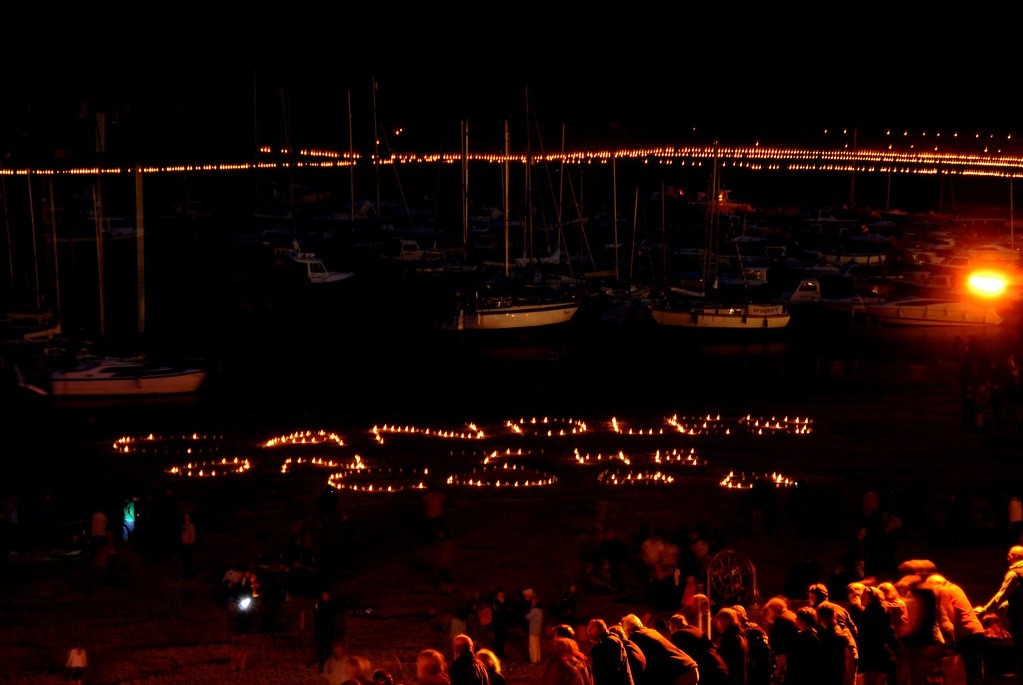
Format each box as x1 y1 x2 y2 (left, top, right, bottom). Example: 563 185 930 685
0 69 1023 419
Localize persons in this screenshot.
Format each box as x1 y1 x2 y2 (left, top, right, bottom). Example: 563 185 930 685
0 481 1023 685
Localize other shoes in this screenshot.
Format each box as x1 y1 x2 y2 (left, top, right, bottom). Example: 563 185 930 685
182 572 192 580
317 652 321 657
327 650 331 655
